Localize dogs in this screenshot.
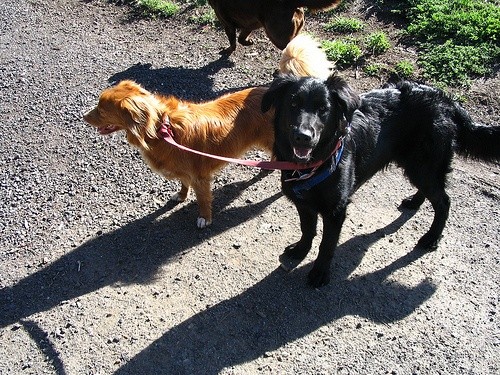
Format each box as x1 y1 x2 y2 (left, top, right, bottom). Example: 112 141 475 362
81 32 337 228
207 0 345 55
258 70 500 291
